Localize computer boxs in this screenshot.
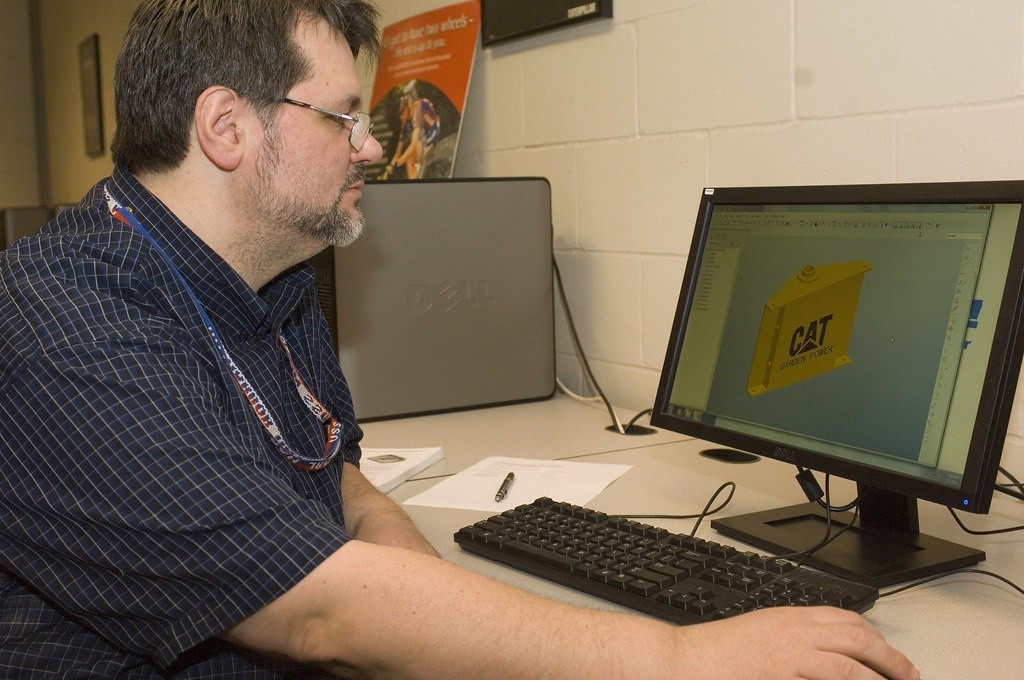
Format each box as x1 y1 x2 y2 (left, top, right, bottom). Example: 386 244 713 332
308 176 555 425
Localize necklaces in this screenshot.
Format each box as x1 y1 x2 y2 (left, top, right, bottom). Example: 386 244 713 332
99 179 346 474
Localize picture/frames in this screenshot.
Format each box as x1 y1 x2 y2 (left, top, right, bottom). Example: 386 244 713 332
77 32 104 158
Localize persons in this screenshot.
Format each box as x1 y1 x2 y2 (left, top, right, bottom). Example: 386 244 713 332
383 80 443 180
0 0 920 679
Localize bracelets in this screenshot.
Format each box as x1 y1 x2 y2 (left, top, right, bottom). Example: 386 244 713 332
390 161 397 168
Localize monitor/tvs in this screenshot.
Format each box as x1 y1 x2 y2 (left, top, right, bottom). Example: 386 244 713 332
651 179 1024 589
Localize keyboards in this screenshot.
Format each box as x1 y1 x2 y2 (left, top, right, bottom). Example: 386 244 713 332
453 495 879 626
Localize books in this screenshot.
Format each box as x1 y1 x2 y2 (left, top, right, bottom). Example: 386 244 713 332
360 446 446 496
365 0 481 181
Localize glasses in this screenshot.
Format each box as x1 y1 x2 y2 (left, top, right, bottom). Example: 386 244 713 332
281 98 374 152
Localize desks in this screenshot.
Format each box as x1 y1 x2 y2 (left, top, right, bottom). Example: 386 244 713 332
357 396 1024 680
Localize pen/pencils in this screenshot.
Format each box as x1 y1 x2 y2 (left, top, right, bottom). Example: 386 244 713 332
495 472 515 502
